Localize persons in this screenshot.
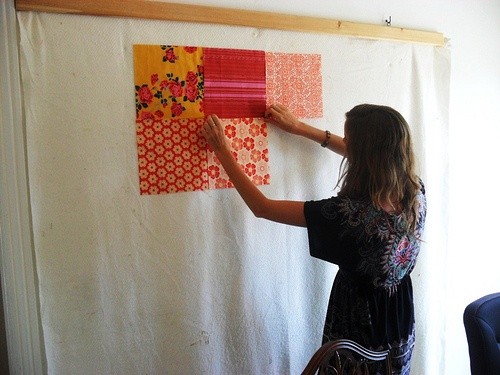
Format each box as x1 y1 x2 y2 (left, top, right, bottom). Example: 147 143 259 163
201 102 426 375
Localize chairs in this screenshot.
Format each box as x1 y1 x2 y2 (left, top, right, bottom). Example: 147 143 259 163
300 338 393 375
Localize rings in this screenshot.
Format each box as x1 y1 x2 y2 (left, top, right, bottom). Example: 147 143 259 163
211 124 216 127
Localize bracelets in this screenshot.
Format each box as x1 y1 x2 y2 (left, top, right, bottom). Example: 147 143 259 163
322 131 331 148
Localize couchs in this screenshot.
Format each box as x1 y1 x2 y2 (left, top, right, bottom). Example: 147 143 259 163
463 292 500 375
